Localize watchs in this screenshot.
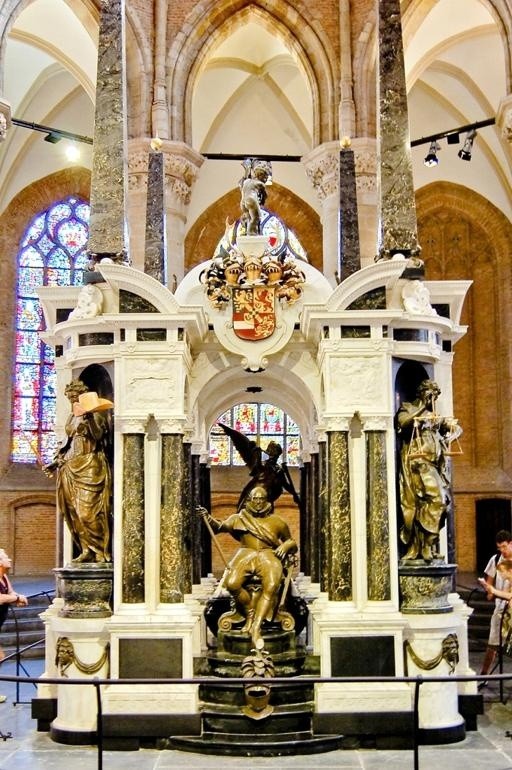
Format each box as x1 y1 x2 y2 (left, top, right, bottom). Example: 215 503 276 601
9 591 19 602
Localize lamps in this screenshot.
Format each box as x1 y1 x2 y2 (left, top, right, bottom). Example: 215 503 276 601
410 116 495 166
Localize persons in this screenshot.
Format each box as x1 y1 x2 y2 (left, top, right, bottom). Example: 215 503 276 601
42 379 114 563
394 379 463 561
477 530 512 691
195 440 298 649
237 158 271 237
0 548 28 703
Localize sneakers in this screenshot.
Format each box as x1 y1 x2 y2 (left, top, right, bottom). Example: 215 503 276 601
478 680 488 691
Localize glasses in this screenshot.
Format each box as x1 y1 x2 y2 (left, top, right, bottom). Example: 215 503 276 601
496 545 509 550
